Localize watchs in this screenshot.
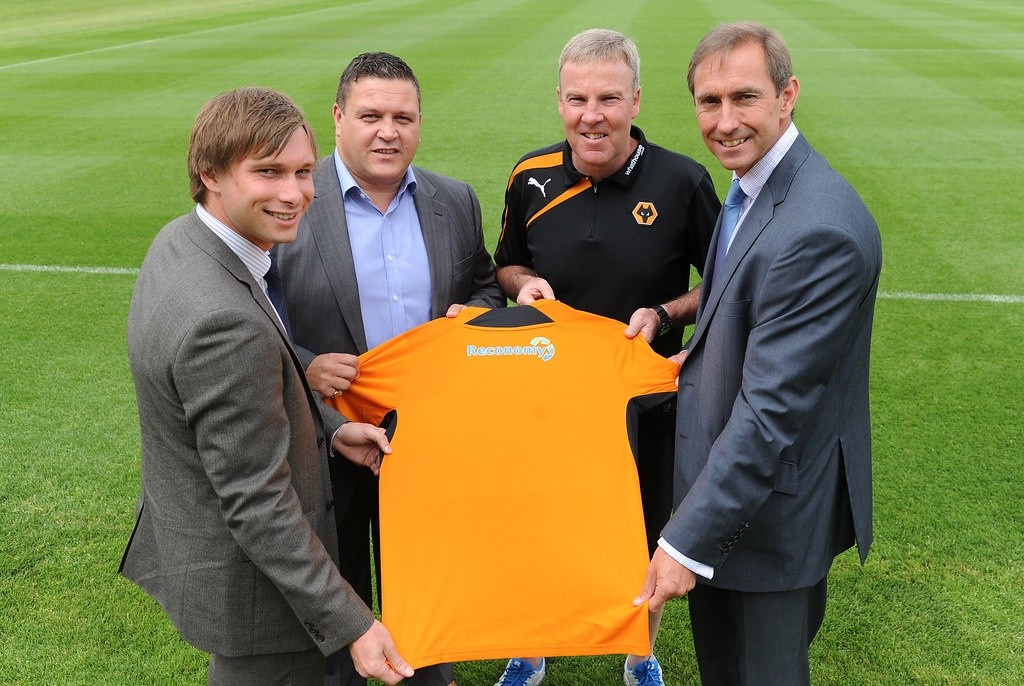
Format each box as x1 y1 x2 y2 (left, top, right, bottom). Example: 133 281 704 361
652 304 672 338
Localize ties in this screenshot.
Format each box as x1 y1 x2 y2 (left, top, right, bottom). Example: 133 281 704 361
710 178 746 303
263 260 295 348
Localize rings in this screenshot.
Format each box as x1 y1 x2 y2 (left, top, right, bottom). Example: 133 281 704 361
330 389 338 398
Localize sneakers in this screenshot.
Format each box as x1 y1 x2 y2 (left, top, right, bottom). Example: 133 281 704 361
491 657 547 686
621 656 665 686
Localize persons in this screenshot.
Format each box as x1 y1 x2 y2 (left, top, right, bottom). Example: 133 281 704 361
494 29 725 686
632 22 883 686
116 89 414 686
277 52 507 686
